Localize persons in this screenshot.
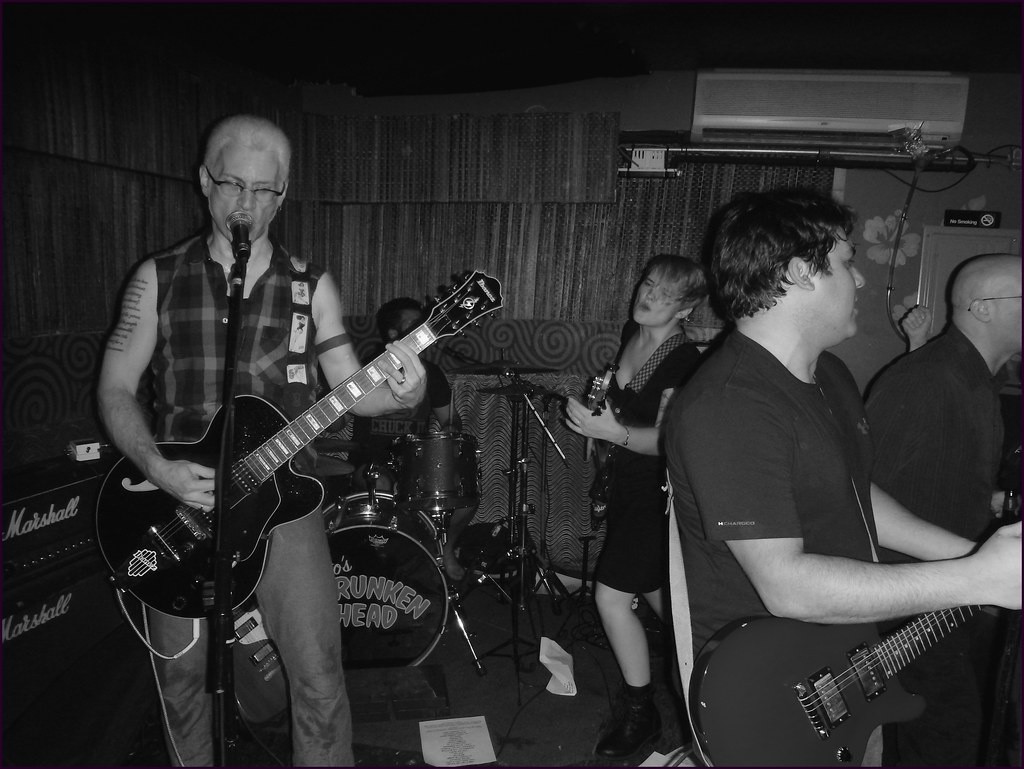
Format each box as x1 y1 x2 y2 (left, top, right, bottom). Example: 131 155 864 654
99 116 429 769
326 297 482 582
563 254 705 758
659 181 1024 769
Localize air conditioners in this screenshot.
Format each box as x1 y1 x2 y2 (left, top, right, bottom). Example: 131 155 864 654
690 65 969 154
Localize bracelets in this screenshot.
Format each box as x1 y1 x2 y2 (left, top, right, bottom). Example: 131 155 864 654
622 426 629 447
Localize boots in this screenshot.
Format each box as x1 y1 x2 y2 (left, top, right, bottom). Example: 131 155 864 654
595 688 662 757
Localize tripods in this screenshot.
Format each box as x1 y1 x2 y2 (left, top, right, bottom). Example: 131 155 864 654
453 371 613 707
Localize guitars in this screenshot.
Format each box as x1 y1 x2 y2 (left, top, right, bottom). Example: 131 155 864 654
228 603 297 725
92 265 507 615
689 605 992 768
586 363 623 531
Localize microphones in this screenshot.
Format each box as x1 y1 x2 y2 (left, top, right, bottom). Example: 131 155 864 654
224 209 253 261
433 340 465 363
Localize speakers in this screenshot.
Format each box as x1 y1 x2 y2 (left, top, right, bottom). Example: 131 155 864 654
1 549 135 769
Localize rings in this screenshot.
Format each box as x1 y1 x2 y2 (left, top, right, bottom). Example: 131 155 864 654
199 505 204 510
576 419 580 425
397 377 406 385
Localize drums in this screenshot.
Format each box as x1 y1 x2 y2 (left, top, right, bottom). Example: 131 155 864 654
389 427 481 519
315 486 452 638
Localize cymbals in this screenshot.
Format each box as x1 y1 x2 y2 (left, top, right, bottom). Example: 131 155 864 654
309 437 359 452
474 378 566 402
447 358 563 375
292 452 355 477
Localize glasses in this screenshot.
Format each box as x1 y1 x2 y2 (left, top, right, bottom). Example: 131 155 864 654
206 166 286 201
